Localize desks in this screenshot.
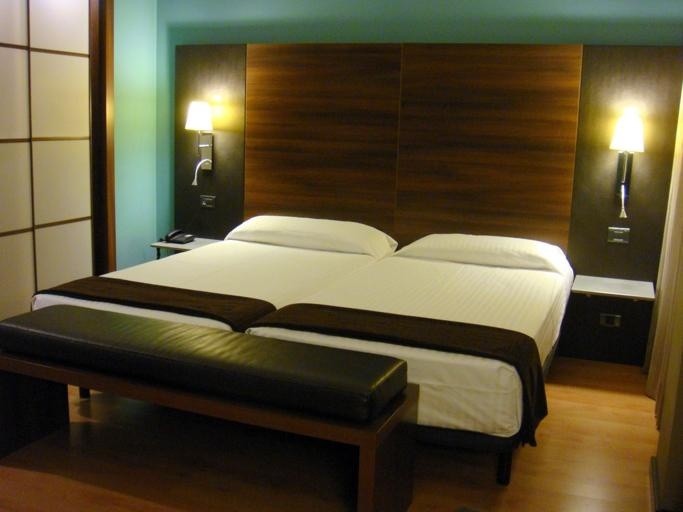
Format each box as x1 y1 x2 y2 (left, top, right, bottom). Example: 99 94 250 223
149 234 221 260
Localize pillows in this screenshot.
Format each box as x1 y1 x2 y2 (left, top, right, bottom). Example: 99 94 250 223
392 231 573 276
224 212 398 261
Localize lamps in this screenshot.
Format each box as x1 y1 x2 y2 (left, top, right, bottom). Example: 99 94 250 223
605 103 648 222
181 99 215 187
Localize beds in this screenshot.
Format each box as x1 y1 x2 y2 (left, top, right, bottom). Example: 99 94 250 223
30 208 576 490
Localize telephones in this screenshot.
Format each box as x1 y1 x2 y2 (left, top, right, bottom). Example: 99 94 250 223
166 229 195 244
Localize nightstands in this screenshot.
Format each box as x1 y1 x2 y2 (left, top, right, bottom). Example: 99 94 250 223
556 274 654 371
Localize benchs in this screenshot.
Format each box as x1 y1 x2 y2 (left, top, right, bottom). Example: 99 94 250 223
0 304 420 510
